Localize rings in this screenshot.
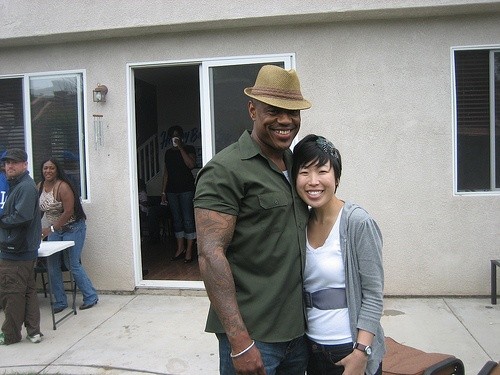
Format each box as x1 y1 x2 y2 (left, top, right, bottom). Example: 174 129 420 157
42 233 43 237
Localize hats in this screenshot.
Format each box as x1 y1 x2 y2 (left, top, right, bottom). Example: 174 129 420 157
244 64 311 110
1 147 30 163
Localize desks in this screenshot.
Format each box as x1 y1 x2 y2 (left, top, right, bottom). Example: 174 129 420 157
37 240 77 329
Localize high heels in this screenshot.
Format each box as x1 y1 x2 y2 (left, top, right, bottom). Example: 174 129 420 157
53 304 68 313
184 255 194 263
79 299 98 310
170 250 186 261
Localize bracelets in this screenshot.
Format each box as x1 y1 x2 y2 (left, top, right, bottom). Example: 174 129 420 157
51 225 58 232
160 192 166 195
230 340 255 357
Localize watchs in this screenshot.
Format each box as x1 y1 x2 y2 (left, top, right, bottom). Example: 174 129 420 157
352 341 372 355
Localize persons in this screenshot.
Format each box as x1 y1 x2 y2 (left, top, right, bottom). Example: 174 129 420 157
160 125 197 263
192 65 312 375
138 179 155 275
0 149 12 213
292 134 387 375
0 148 45 345
36 157 99 314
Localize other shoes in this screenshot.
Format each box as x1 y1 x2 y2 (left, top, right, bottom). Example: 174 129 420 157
0 333 15 345
26 335 42 343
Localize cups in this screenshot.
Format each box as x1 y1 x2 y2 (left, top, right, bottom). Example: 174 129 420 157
172 137 179 147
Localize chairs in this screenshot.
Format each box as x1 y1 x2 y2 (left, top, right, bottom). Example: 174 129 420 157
40 234 82 297
370 333 500 375
